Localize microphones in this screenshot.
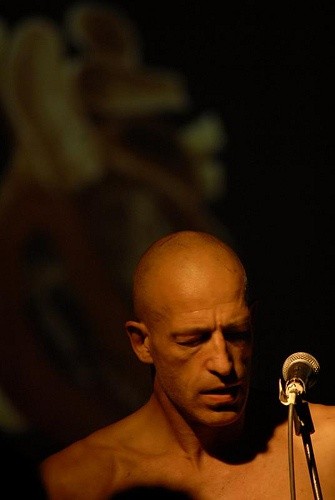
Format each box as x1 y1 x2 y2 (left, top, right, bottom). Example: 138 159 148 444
282 351 319 395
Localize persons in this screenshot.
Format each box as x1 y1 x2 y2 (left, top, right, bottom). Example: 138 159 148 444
36 230 334 499
